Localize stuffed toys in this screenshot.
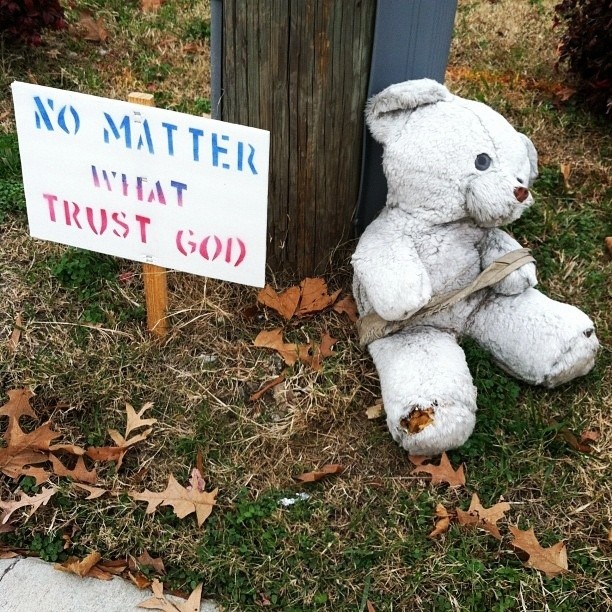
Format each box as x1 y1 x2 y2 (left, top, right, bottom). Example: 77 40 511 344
351 78 600 456
